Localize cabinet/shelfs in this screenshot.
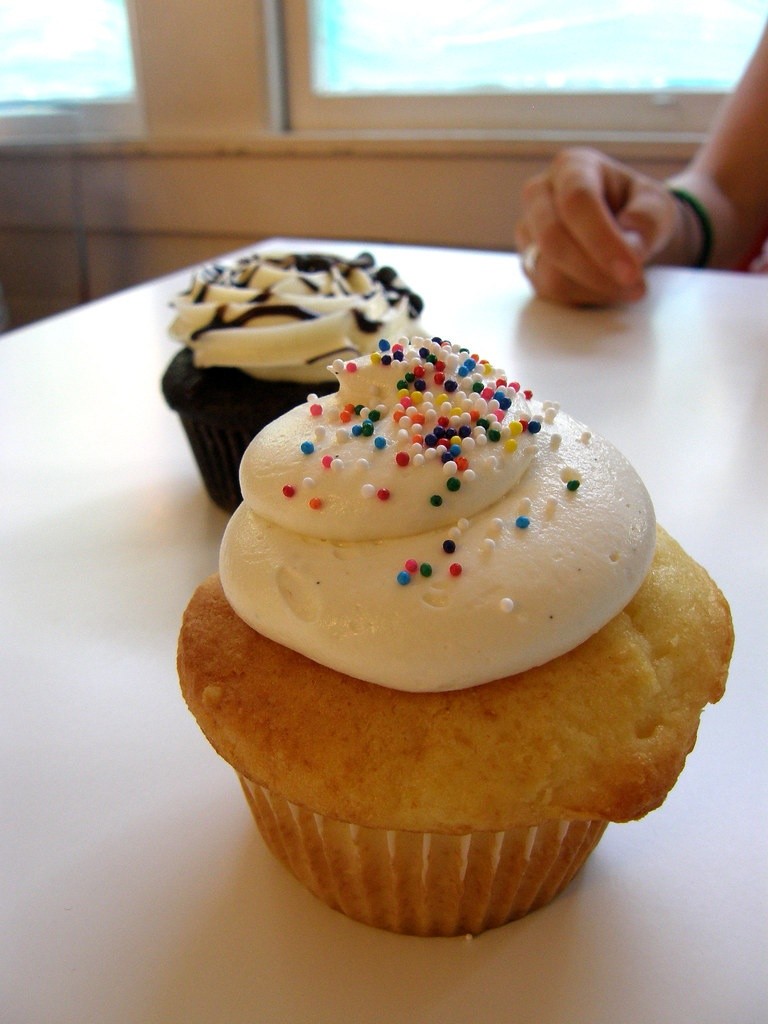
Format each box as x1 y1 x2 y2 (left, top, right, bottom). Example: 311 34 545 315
0 124 768 335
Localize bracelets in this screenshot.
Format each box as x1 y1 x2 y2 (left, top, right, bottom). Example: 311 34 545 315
664 182 716 267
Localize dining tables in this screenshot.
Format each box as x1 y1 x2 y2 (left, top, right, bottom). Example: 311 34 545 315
0 238 764 1024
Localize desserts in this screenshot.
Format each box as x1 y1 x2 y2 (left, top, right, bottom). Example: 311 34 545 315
177 334 735 940
159 251 423 510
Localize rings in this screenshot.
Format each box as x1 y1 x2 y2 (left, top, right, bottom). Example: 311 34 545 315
520 241 542 283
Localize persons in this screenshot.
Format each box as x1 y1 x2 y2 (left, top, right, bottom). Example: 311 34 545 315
517 21 767 316
